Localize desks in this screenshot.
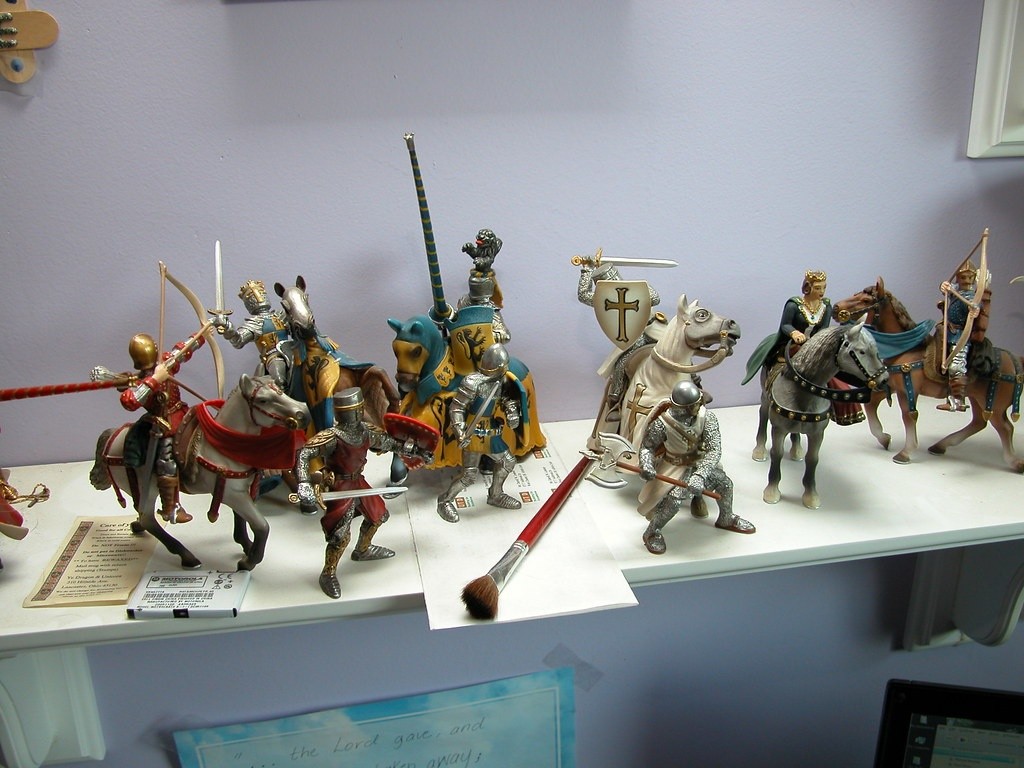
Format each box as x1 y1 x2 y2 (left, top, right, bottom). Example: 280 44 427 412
1 390 1024 768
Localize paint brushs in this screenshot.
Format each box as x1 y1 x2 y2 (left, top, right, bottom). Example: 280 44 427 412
461 456 595 622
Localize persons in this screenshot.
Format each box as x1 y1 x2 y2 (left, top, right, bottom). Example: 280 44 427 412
455 275 511 343
436 343 521 521
638 381 756 555
120 325 215 522
578 256 712 419
936 258 992 411
216 279 290 391
776 267 867 426
296 387 435 599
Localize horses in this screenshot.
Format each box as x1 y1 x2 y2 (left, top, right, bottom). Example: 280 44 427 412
588 294 742 468
89 373 313 569
383 313 545 497
832 276 1024 475
752 311 890 510
253 275 401 419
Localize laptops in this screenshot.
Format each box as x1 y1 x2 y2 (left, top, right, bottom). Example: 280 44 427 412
873 678 1023 768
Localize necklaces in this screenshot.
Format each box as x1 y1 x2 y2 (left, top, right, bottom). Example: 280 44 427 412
804 301 821 320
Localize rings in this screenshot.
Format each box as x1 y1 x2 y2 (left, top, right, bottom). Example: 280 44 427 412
797 338 799 340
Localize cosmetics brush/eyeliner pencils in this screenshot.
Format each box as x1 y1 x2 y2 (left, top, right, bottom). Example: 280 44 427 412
458 448 597 623
